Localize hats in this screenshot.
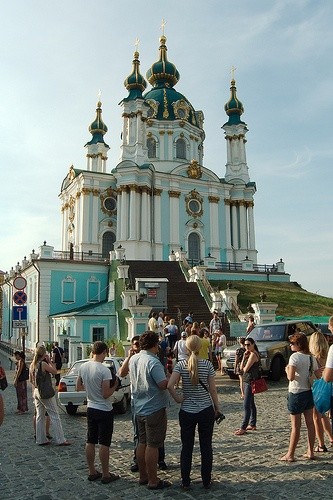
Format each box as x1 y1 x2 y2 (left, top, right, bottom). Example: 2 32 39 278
180 332 188 337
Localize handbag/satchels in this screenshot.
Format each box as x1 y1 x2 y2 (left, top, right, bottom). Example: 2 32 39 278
250 377 268 393
17 360 29 381
175 360 179 365
311 375 333 415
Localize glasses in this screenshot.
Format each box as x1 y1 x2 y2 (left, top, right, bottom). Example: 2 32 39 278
289 341 296 345
133 342 139 346
244 344 250 347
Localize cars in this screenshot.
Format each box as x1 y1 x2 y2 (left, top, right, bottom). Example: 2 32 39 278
57 355 130 415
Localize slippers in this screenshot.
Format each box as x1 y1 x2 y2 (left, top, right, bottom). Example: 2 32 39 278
246 426 256 430
138 476 171 489
277 455 294 462
235 430 247 434
307 451 314 460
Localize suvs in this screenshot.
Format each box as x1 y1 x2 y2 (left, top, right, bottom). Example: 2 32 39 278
220 319 321 382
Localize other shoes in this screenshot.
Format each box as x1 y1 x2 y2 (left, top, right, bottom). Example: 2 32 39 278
88 469 103 480
159 460 166 470
46 434 52 438
58 440 71 445
33 433 36 439
204 479 214 489
179 480 189 489
101 473 119 483
131 465 138 471
313 444 328 451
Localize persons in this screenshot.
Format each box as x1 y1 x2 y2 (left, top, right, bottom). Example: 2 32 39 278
236 338 262 435
294 328 306 336
245 315 255 337
308 316 333 452
167 335 220 488
29 345 71 445
77 341 121 483
119 331 173 489
39 342 64 386
0 362 8 426
13 350 29 414
234 337 245 398
149 311 226 389
279 332 318 461
